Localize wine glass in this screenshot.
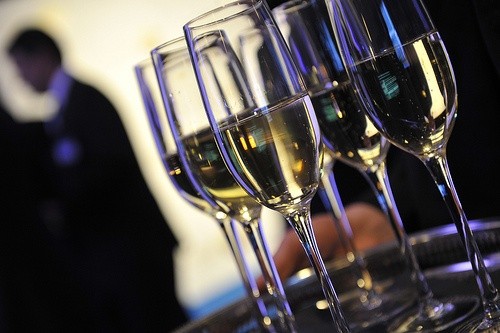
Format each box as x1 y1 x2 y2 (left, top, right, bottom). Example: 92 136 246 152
133 1 500 333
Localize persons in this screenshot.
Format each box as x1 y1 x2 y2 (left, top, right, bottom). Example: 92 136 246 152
251 0 500 292
0 26 191 333
